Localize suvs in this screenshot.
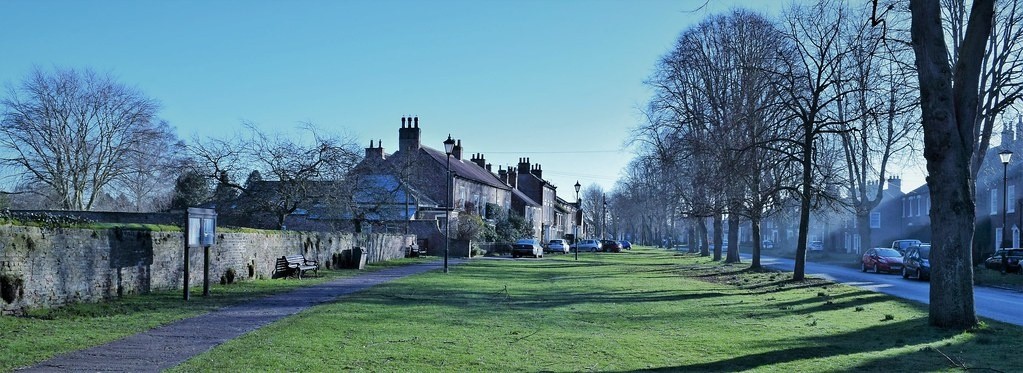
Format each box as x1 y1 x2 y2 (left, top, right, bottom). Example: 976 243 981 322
901 243 932 280
569 238 604 254
808 239 825 252
985 246 1023 272
597 239 623 252
891 240 920 257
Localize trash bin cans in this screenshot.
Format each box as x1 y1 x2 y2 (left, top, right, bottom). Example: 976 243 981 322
352 246 368 270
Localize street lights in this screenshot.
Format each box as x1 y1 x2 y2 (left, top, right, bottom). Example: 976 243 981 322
574 180 582 260
443 133 457 274
603 201 608 242
997 149 1013 275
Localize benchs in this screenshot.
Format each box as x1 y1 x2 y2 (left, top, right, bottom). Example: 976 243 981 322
410 245 427 258
282 254 319 281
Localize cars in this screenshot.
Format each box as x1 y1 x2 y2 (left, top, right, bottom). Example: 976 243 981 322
861 247 906 274
1016 259 1023 273
618 240 632 250
708 242 730 251
510 238 544 259
546 238 570 256
763 240 775 249
